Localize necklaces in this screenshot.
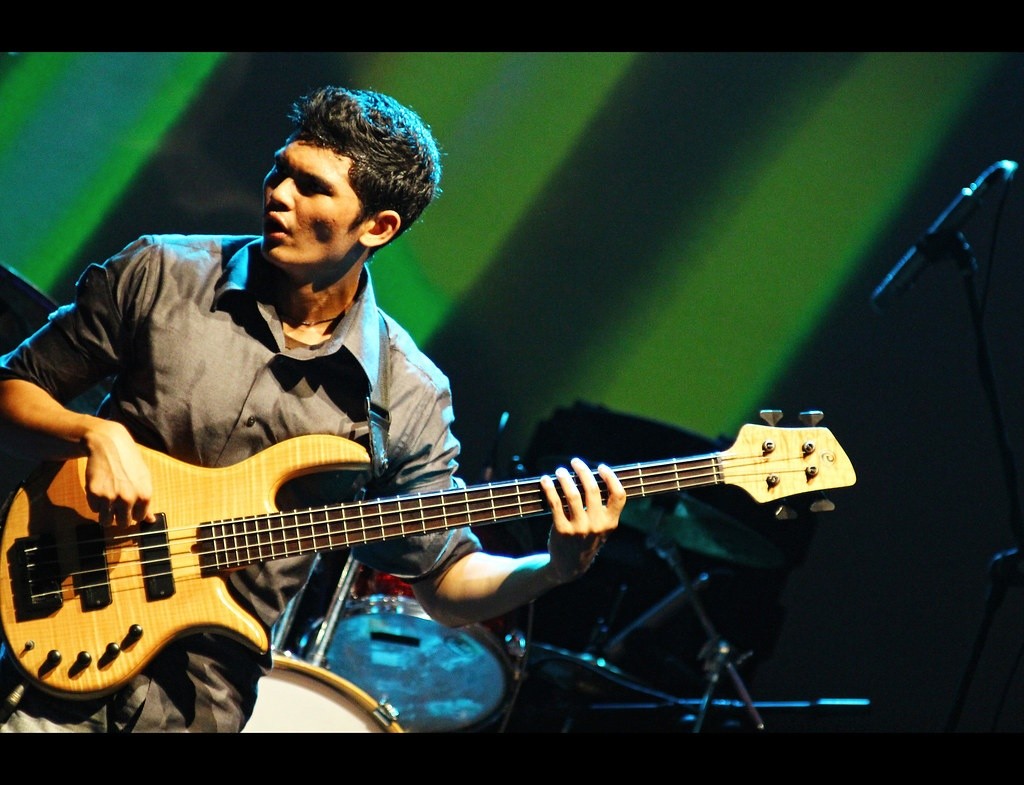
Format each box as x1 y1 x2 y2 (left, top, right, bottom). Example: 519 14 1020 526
284 310 338 326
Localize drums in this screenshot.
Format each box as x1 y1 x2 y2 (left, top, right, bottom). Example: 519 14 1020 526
300 564 521 730
241 652 406 734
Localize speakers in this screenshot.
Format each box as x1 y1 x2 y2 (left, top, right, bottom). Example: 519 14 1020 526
479 401 825 735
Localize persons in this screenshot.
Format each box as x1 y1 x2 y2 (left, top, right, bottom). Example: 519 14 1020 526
0 85 626 733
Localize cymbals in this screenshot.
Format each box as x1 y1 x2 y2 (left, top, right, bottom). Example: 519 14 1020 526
616 488 791 574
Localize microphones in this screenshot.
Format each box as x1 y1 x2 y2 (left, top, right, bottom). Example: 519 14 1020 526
871 160 1016 308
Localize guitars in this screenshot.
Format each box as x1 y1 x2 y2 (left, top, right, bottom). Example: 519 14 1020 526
0 408 856 736
